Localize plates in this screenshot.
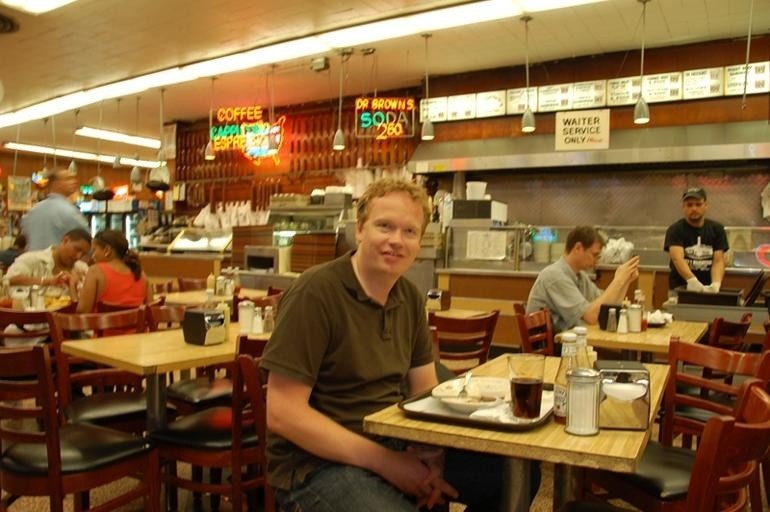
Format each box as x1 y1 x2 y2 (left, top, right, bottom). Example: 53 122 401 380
647 322 668 328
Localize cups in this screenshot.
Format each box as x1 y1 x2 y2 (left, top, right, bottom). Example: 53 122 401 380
465 180 488 201
505 351 545 420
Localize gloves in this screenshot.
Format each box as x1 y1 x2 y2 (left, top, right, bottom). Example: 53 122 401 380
689 278 721 294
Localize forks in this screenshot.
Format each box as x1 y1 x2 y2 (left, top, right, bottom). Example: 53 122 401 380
456 371 474 400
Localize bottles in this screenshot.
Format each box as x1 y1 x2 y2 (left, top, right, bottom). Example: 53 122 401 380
573 325 591 371
552 331 580 425
607 288 648 333
0 269 46 312
205 265 276 345
563 365 599 438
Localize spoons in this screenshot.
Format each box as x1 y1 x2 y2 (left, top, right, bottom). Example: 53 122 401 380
430 392 505 402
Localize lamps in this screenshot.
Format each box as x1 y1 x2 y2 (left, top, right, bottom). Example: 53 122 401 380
421 34 435 141
268 64 279 156
520 16 536 133
333 50 346 150
204 76 217 161
9 87 170 193
633 0 651 125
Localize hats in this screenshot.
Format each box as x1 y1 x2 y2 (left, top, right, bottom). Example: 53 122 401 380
682 186 706 200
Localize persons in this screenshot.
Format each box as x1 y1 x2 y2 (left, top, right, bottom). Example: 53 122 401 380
23 169 94 258
76 230 159 400
262 179 542 512
524 225 642 358
661 187 729 303
0 236 27 267
4 228 96 431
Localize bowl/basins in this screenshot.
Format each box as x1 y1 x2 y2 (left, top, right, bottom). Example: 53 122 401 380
429 376 510 413
